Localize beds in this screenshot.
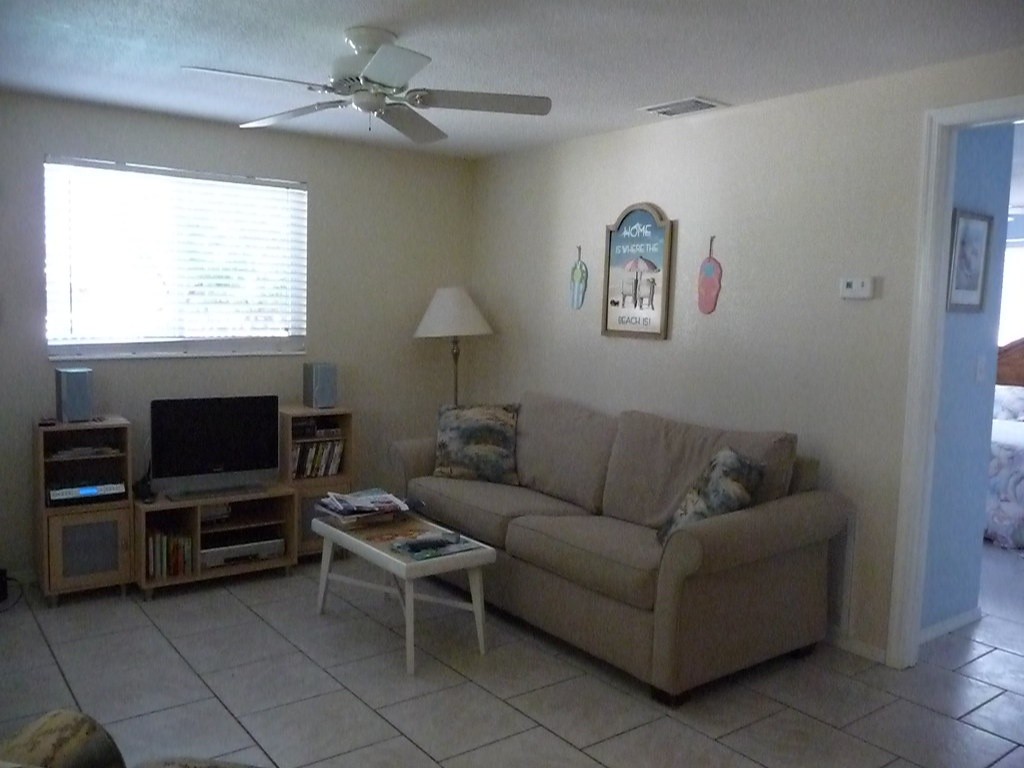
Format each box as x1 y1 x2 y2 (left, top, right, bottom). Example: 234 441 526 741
984 338 1024 552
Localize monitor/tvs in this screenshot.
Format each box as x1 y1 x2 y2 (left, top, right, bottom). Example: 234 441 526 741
150 395 280 492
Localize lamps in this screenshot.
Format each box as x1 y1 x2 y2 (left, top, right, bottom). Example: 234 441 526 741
414 285 493 405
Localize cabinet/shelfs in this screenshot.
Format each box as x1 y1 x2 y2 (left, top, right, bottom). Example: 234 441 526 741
30 408 360 604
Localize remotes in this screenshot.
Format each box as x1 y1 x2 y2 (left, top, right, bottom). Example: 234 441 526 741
144 492 157 504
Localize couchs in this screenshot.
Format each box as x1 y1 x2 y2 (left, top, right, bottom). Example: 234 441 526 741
386 399 846 707
0 709 253 768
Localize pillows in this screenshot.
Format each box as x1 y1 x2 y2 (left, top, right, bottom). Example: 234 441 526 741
433 401 522 488
656 443 764 546
992 385 1023 421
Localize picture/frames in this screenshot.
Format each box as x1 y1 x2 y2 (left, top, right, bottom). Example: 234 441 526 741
601 201 674 340
945 205 995 315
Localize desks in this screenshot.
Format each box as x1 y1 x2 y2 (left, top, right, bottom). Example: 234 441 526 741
309 512 499 673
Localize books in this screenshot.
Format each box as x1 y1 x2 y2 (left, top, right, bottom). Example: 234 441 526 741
148 530 192 583
392 532 482 561
290 427 345 479
312 484 411 529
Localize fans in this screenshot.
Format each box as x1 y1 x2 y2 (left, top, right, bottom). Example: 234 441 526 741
178 24 554 149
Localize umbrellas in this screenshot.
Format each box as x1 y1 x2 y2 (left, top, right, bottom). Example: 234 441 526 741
624 255 661 310
